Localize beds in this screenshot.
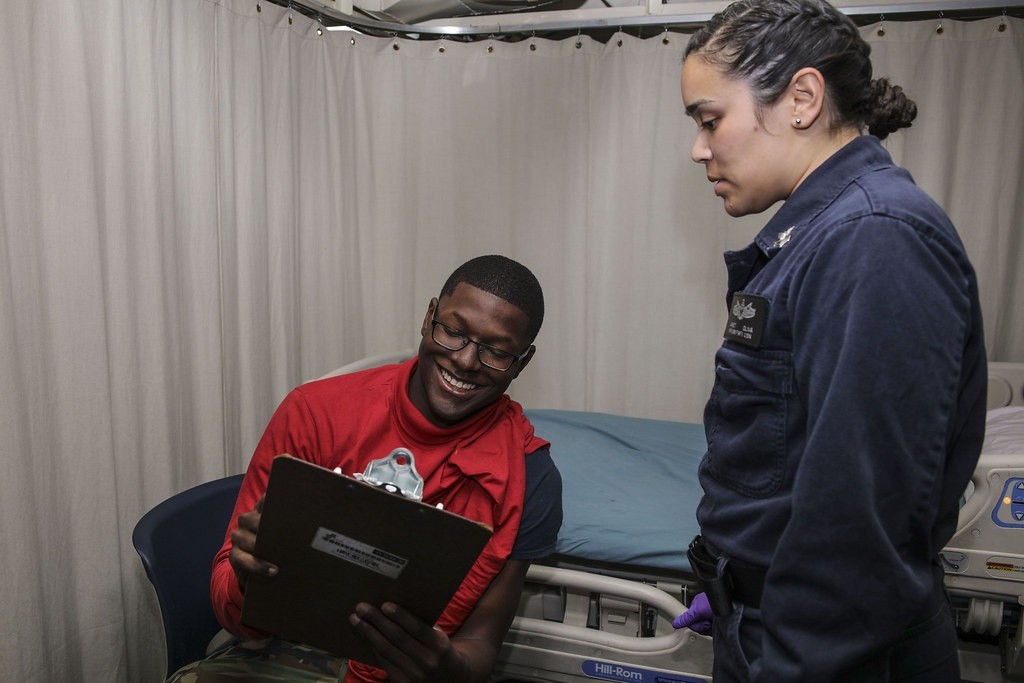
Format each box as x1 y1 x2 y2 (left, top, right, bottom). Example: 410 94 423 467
488 362 1024 683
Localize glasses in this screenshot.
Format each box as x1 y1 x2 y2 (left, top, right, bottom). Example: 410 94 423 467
431 303 533 372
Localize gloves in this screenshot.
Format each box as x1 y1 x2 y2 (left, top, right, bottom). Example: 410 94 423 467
672 590 713 637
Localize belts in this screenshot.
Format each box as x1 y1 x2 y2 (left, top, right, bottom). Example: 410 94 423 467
685 536 766 616
234 634 346 676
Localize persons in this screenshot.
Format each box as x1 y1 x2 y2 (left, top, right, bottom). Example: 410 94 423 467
165 255 563 683
672 0 988 683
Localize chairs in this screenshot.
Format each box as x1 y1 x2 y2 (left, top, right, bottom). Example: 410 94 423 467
132 469 246 680
